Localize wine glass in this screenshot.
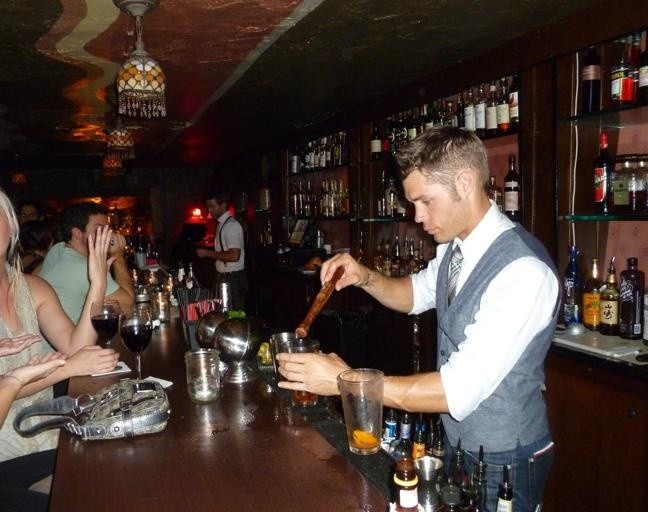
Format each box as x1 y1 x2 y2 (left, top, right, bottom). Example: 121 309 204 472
90 299 123 372
116 306 153 382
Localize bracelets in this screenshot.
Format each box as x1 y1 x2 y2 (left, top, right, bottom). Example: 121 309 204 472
2 371 24 386
357 262 374 288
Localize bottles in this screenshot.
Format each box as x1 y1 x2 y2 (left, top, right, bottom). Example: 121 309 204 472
365 72 518 161
558 242 648 344
127 264 199 324
386 408 546 512
276 243 291 264
286 130 347 172
579 40 648 112
373 152 521 217
592 127 647 218
135 236 146 270
373 231 427 280
288 176 350 215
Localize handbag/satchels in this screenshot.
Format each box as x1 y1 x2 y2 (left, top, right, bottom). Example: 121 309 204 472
14 379 170 440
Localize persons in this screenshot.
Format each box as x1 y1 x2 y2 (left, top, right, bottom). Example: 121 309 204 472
1 191 120 511
276 125 561 512
196 192 248 319
1 334 67 430
39 202 137 322
8 194 58 276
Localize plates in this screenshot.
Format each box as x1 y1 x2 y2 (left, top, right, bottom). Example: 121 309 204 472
297 267 316 275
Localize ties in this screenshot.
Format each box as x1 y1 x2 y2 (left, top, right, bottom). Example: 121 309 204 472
445 246 463 307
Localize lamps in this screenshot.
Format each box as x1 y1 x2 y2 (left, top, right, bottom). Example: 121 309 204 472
105 84 138 158
112 0 168 119
100 140 124 177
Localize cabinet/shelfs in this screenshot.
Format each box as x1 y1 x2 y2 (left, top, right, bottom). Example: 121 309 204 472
289 131 357 265
545 21 647 511
358 60 545 288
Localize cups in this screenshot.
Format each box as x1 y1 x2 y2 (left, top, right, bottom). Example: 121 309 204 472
240 192 247 211
183 347 222 404
281 339 321 411
323 244 332 254
336 366 385 458
255 343 273 371
